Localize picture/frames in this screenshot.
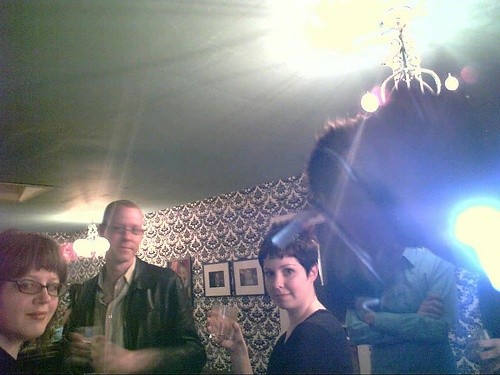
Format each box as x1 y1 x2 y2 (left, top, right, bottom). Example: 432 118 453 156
203 262 231 298
231 259 265 295
168 257 192 298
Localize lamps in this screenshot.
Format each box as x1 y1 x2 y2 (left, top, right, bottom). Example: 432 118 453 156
360 3 460 112
72 221 110 257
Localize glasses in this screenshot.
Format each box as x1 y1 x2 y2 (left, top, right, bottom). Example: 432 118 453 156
104 223 146 235
1 276 72 297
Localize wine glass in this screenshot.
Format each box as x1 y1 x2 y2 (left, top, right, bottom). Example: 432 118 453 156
469 329 499 375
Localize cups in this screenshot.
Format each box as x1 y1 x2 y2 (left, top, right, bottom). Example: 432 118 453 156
77 325 104 344
210 304 239 339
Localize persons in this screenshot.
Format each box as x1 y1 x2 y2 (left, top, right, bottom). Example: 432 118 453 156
0 227 68 375
62 199 208 375
346 230 500 375
207 219 353 375
